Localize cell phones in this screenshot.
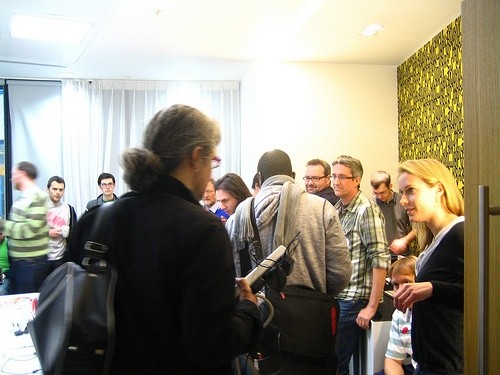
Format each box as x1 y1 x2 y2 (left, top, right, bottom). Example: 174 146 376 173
384 289 411 307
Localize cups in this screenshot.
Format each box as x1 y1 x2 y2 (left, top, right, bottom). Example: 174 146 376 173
57 226 70 238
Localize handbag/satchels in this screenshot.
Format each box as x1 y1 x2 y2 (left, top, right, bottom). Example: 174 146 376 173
261 285 340 356
27 202 118 375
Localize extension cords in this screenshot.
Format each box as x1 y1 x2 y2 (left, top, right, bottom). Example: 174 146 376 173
234 244 288 297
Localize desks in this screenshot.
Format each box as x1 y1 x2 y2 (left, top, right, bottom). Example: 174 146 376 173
0 293 44 375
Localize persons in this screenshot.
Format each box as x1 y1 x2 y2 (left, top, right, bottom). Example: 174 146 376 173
383 255 418 375
367 170 417 321
326 155 389 375
302 158 340 206
224 149 353 375
0 161 51 297
45 176 78 275
392 157 464 374
214 172 252 225
249 172 262 197
61 104 264 375
200 178 229 223
84 172 118 213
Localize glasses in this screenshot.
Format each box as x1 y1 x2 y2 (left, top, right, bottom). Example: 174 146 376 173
303 176 326 182
327 175 356 181
211 157 220 168
372 189 388 196
102 183 114 187
